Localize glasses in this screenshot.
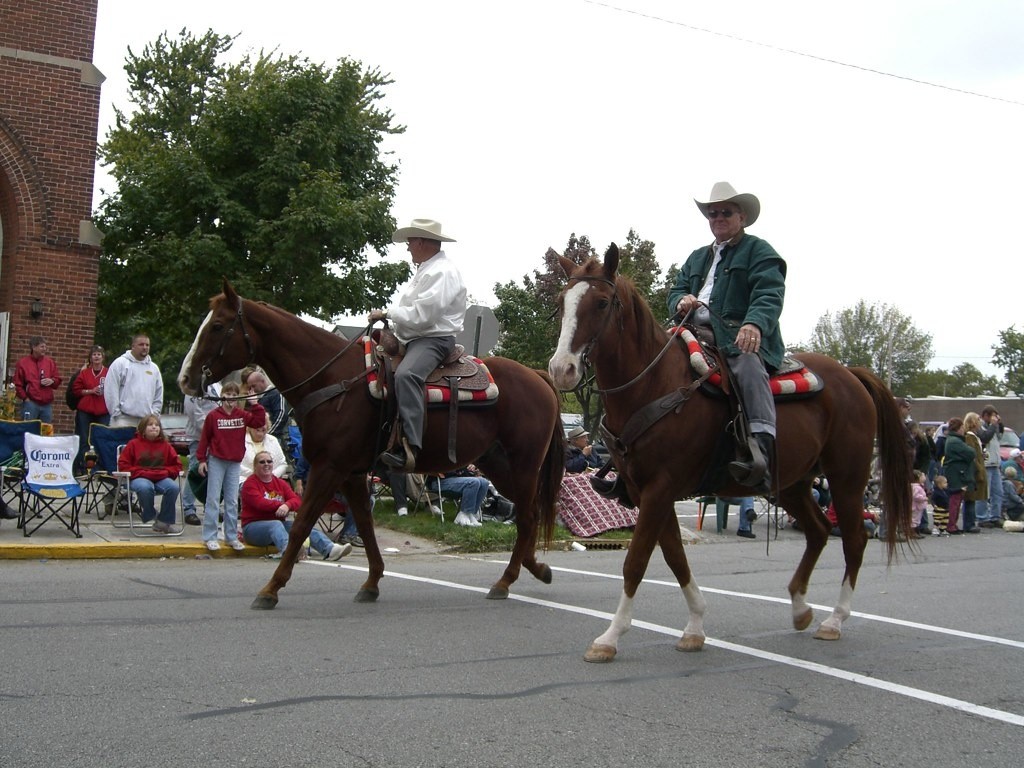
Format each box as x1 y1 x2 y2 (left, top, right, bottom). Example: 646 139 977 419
259 459 273 464
707 208 740 218
404 240 409 246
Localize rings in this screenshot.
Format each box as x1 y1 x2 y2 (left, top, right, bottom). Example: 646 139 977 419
750 337 756 340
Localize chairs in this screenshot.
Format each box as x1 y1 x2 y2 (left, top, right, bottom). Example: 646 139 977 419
0 418 460 539
697 494 788 530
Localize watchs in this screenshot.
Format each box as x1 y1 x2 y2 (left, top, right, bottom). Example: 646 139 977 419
382 309 388 319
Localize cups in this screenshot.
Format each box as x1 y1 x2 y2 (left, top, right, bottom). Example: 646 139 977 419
100 385 104 395
571 542 586 551
24 412 30 421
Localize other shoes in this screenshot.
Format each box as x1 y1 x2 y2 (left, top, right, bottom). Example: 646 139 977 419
185 514 201 525
977 522 995 527
0 498 20 519
381 446 424 473
730 459 772 494
990 520 1003 526
737 529 756 537
745 509 757 522
949 530 964 535
152 520 179 533
105 504 118 515
153 509 160 521
964 527 982 532
118 502 142 511
921 528 932 534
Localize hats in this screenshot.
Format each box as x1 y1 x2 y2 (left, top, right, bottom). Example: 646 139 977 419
694 181 760 227
566 426 590 438
392 218 457 243
1009 447 1021 457
947 417 963 431
964 411 980 429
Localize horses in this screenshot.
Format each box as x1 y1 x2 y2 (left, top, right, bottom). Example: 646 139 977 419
175 278 564 610
547 241 927 665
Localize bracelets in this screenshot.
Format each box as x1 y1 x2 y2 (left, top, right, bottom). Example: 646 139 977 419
85 390 89 395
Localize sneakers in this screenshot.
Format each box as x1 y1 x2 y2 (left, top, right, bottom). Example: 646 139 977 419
339 536 365 546
398 507 407 516
454 510 482 527
430 505 444 514
225 540 245 550
206 540 220 551
328 542 353 561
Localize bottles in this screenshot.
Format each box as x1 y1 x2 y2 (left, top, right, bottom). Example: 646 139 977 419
7 383 16 393
41 369 44 387
89 446 94 455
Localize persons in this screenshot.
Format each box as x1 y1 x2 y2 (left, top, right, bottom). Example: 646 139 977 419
718 397 1024 543
367 219 467 471
118 415 182 533
72 345 108 481
104 332 163 516
0 495 21 519
564 425 605 474
182 369 352 563
13 336 60 424
589 181 786 509
292 455 489 547
